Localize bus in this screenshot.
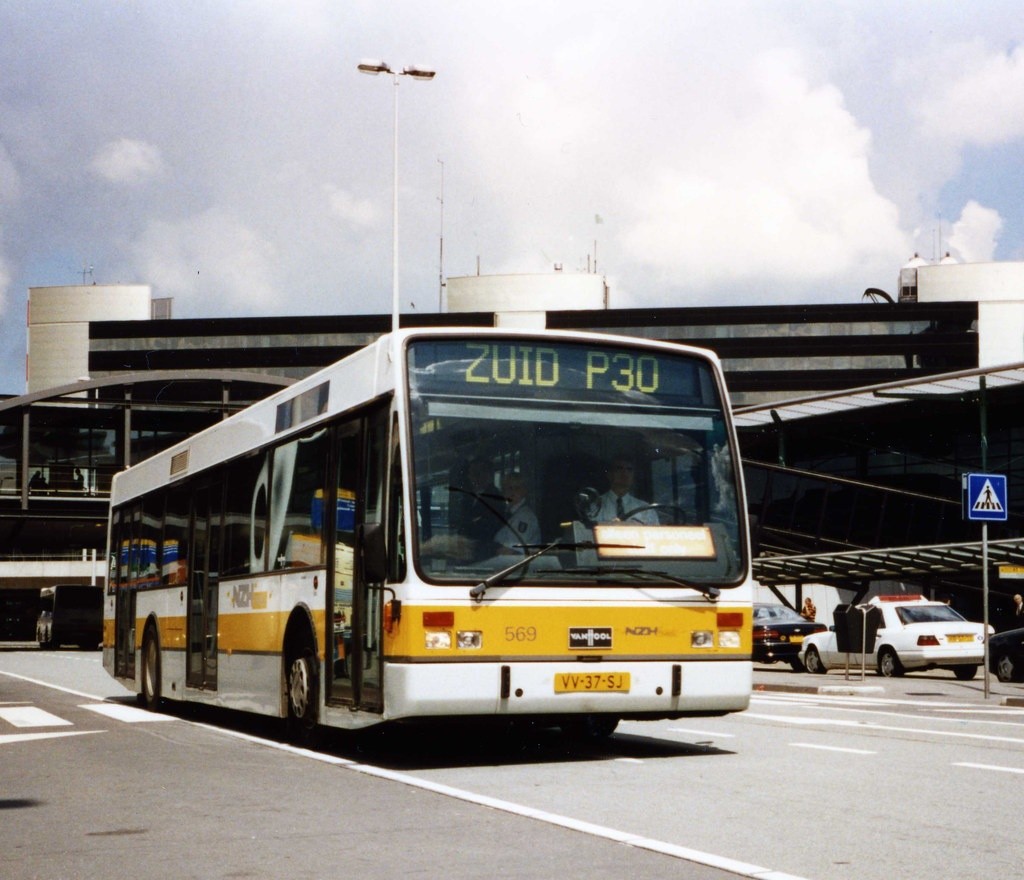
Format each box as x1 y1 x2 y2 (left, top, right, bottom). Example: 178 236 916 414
100 329 752 747
36 586 104 650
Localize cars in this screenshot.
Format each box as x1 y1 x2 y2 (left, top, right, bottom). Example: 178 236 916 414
798 595 996 681
751 602 827 665
982 628 1024 682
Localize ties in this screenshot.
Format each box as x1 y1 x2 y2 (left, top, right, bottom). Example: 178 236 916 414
617 497 624 517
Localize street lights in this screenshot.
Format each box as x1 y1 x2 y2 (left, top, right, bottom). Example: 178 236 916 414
357 56 435 337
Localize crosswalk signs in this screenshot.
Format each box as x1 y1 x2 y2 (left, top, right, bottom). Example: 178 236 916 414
970 474 1009 524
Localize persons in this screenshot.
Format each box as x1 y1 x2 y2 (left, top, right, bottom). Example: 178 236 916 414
799 597 816 622
458 456 541 558
1012 593 1024 630
28 471 48 496
590 454 660 526
75 468 84 490
946 593 956 609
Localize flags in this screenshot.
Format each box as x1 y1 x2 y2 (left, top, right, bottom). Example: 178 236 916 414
595 214 604 226
